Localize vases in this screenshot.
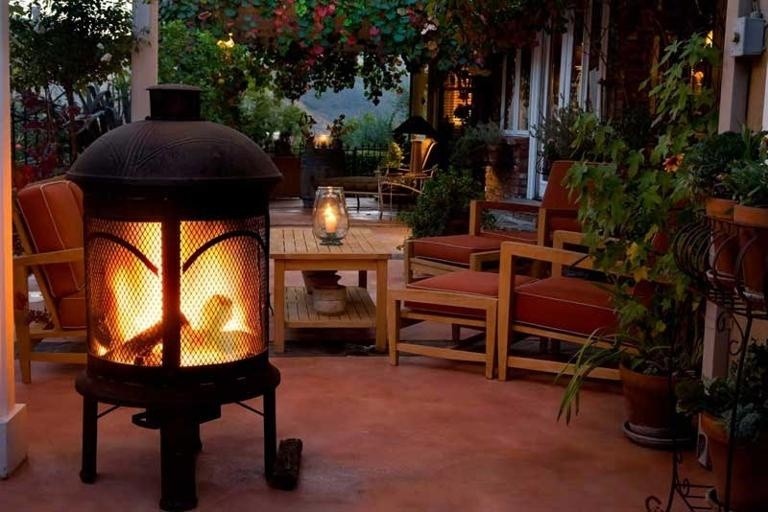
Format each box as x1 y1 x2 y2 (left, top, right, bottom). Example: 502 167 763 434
299 269 348 315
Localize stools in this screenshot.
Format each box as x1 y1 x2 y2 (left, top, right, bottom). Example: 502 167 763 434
382 232 540 381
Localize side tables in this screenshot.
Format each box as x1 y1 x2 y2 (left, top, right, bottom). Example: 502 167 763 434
270 225 393 356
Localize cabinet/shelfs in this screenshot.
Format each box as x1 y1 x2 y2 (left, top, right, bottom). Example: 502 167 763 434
644 210 766 512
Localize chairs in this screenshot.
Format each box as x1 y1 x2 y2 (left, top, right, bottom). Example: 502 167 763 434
471 159 675 388
7 176 86 382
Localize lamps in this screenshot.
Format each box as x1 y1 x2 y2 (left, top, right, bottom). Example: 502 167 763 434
310 185 350 247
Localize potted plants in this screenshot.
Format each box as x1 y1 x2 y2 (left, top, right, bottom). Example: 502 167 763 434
546 328 695 448
697 368 766 509
683 133 768 302
